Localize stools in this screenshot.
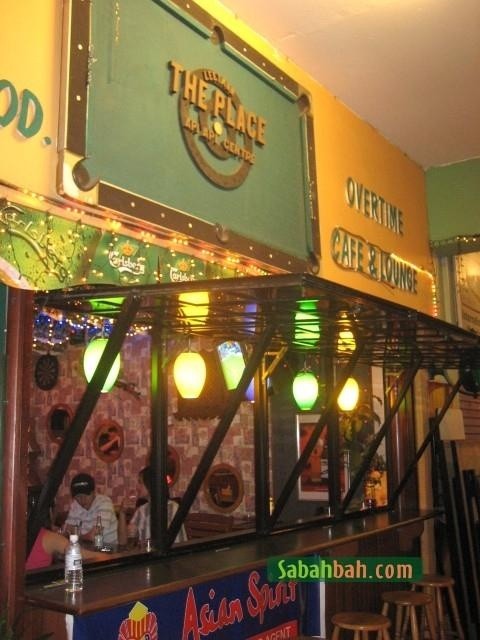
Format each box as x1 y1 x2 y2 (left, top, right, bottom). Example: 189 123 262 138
329 610 397 640
410 571 466 640
379 590 436 639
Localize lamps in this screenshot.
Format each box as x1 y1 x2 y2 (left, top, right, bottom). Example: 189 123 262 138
82 316 123 393
173 327 207 404
216 339 247 391
291 351 320 412
338 365 360 415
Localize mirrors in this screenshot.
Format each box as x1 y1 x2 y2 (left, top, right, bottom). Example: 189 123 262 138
201 463 244 514
139 442 184 487
93 419 125 463
43 400 74 448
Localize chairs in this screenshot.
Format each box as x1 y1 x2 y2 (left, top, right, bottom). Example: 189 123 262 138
183 511 237 540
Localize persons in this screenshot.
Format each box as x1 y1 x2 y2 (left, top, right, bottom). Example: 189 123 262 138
60 473 118 548
117 466 189 548
24 484 111 571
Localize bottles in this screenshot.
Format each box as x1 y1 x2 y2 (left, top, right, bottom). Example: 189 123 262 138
63 534 84 592
95 513 104 550
